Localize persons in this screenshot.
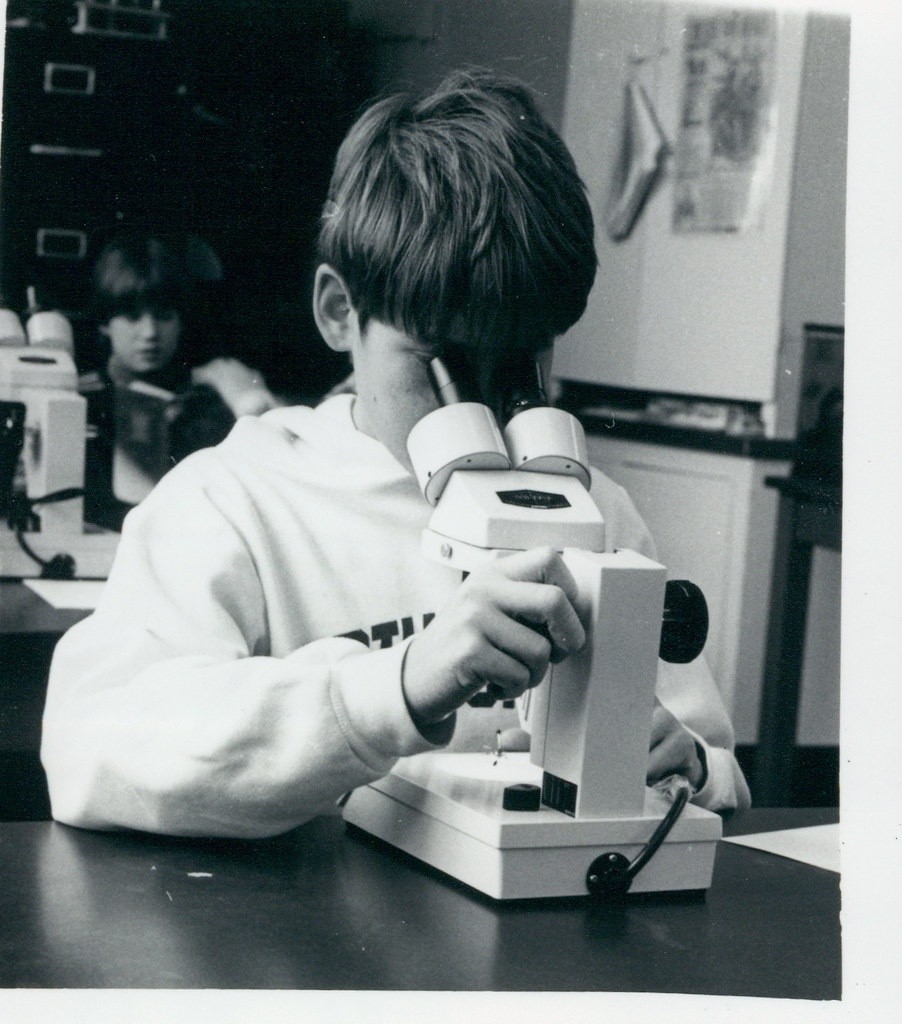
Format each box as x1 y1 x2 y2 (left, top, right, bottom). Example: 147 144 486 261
41 75 752 837
22 238 277 532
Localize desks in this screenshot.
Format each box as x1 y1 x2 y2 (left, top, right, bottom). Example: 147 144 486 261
1 805 840 1000
747 474 842 810
